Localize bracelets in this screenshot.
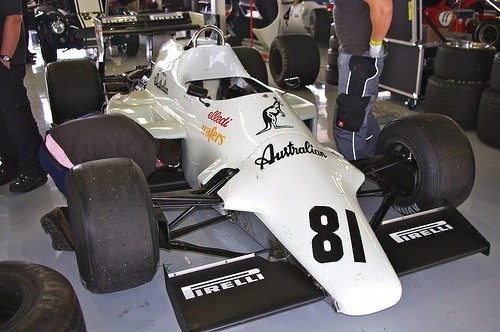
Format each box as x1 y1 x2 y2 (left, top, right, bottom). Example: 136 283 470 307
371 38 382 46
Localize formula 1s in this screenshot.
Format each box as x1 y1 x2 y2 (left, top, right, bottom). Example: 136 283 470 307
36 8 492 332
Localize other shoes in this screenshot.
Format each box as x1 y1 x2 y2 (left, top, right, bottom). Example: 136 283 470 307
26 51 36 64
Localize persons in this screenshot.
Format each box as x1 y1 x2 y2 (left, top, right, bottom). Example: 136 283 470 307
0 1 48 193
21 1 37 64
333 1 394 162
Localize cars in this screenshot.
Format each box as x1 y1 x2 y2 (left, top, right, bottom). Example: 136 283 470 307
27 0 140 64
422 0 500 52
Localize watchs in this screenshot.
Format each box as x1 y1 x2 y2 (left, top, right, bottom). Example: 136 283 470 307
0 55 12 63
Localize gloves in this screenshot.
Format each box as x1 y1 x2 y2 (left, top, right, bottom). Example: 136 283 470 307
369 41 388 59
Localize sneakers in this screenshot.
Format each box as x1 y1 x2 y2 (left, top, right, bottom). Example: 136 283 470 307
0 162 19 185
9 174 48 193
